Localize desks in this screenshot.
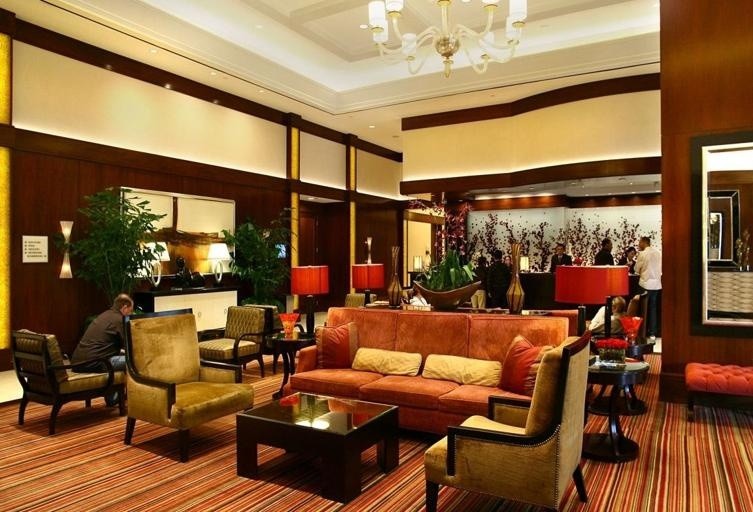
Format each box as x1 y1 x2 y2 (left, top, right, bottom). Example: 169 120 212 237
584 334 651 463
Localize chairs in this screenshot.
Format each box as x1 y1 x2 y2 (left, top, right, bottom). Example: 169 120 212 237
424 329 590 510
197 304 316 384
10 327 127 435
123 308 255 461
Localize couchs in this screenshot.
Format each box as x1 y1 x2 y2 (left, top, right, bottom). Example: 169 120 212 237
284 306 570 435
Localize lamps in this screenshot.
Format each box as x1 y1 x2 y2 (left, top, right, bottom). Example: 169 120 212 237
365 235 372 265
349 261 386 306
599 266 631 336
413 251 421 271
205 242 230 283
518 253 530 273
551 259 610 332
290 261 329 337
357 0 531 83
58 217 73 283
142 241 169 286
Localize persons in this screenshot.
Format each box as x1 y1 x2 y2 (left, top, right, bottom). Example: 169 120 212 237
593 239 614 265
550 244 572 274
633 237 663 338
618 247 636 275
410 273 427 306
589 296 626 340
470 250 511 308
70 293 134 406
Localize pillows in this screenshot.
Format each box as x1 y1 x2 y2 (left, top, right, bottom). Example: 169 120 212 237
352 347 422 377
422 353 503 387
315 321 359 369
500 334 554 398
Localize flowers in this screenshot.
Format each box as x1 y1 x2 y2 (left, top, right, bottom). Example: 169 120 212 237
412 193 472 261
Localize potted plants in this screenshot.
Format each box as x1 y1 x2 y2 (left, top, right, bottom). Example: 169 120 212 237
413 246 481 311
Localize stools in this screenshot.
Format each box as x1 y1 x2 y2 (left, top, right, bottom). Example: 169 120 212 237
682 360 752 425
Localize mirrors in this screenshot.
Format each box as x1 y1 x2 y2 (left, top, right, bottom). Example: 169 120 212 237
688 129 751 339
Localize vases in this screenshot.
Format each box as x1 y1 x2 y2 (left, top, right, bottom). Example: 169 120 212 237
506 238 526 314
383 244 404 306
